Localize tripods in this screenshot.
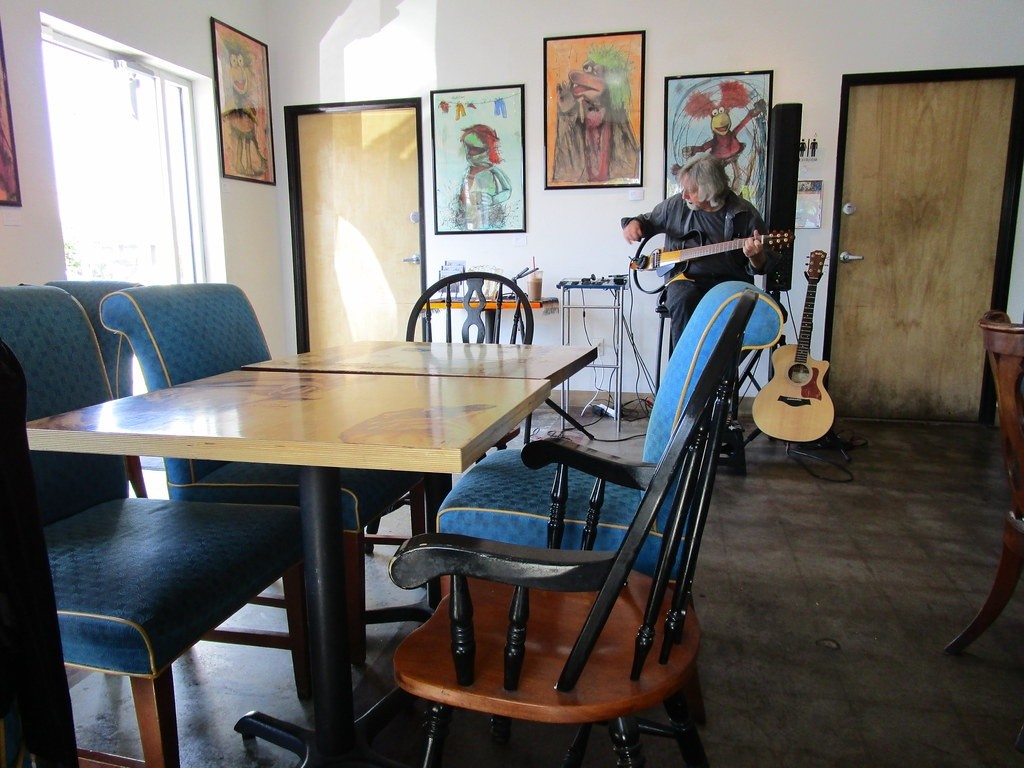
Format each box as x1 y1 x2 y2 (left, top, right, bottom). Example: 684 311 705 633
735 292 840 446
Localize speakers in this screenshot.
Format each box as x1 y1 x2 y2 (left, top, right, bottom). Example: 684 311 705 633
761 103 803 292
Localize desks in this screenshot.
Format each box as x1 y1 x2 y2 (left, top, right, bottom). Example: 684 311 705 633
23 342 600 768
420 294 560 344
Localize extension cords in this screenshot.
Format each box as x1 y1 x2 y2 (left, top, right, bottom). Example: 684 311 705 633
599 403 615 418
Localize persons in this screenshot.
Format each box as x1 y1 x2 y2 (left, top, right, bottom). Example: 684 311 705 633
620 155 770 359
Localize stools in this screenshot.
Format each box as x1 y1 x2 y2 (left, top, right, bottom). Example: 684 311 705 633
654 287 748 477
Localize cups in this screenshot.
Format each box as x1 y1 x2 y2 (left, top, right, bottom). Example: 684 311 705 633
526 270 543 301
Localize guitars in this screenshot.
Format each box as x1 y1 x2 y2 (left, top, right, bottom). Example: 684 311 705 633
632 228 796 295
752 250 835 443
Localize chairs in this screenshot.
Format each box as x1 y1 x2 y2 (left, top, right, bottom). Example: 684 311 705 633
433 280 787 734
404 271 536 452
96 281 451 670
942 311 1024 755
0 284 312 768
19 278 149 499
392 286 762 768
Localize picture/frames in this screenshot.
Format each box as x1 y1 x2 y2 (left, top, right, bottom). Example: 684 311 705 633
209 15 276 186
430 83 527 235
543 29 647 191
663 70 774 231
0 16 22 207
795 179 824 229
